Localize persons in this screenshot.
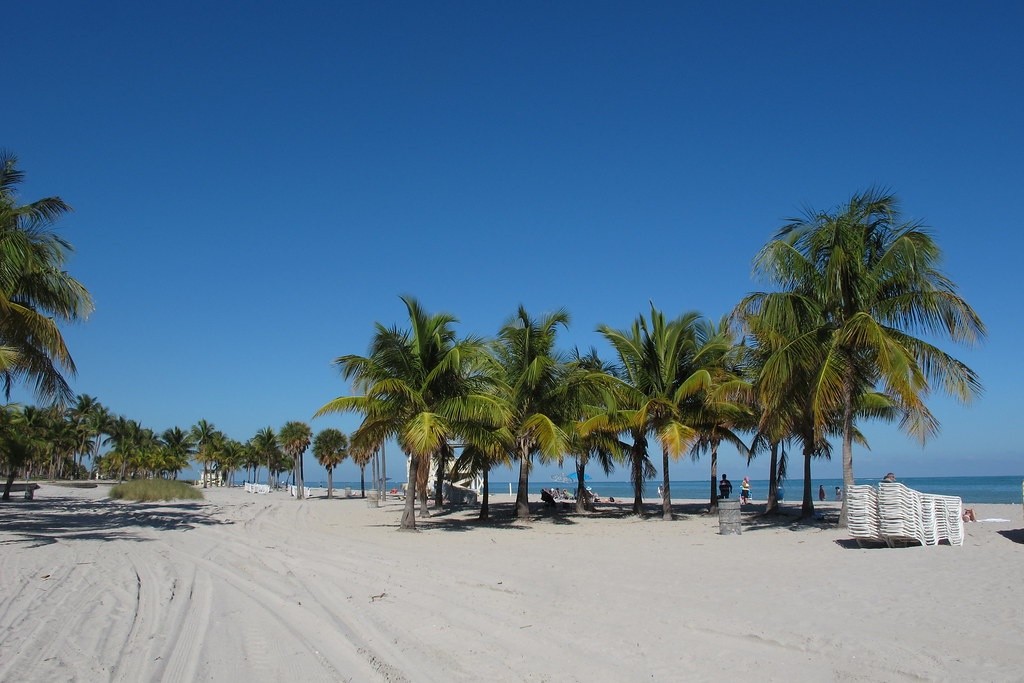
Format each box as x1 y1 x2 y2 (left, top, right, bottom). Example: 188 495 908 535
657 484 664 504
819 485 826 501
883 473 896 483
742 477 751 504
835 487 842 500
541 485 599 500
719 474 732 498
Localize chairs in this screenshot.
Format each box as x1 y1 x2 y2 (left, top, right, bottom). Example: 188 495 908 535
583 487 594 502
846 482 964 546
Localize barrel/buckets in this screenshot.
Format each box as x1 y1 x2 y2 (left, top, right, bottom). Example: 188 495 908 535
367 489 378 509
345 487 351 497
717 499 742 535
